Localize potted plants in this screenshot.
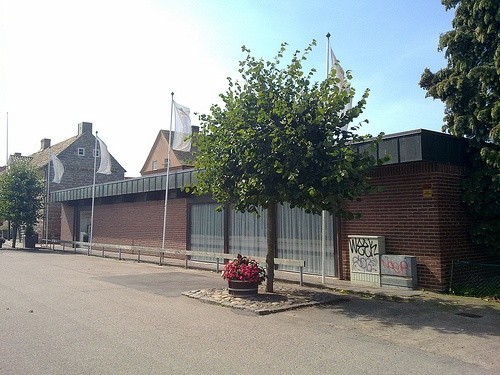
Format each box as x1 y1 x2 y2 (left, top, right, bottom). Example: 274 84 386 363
222 253 266 297
23 225 35 248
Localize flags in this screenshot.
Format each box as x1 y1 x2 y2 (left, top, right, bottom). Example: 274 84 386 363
94 139 112 174
51 154 65 182
330 47 352 139
172 99 191 152
